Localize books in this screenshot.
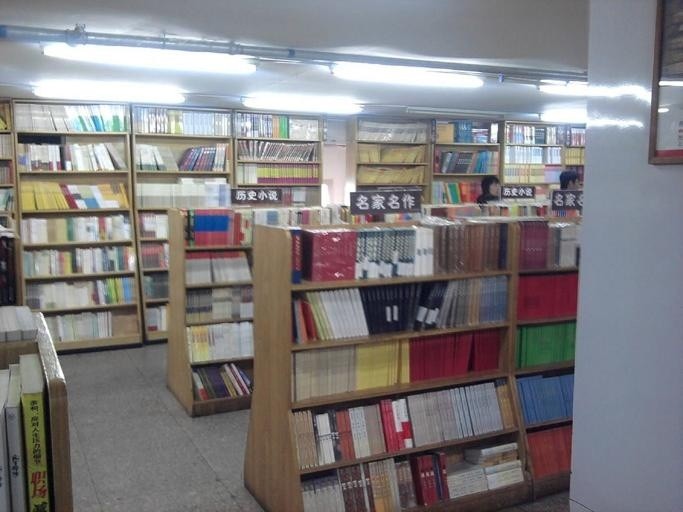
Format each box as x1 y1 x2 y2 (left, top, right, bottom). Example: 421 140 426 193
14 103 138 342
0 102 14 229
134 106 320 208
139 209 581 511
355 116 586 218
1 306 50 512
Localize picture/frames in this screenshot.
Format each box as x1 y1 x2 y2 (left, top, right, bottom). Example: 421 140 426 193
648 0 683 165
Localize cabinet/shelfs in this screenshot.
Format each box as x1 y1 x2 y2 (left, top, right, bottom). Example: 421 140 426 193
243 223 578 511
166 203 582 418
131 102 234 340
0 98 22 308
503 122 584 205
11 100 142 346
346 115 432 205
431 117 503 205
232 109 324 206
